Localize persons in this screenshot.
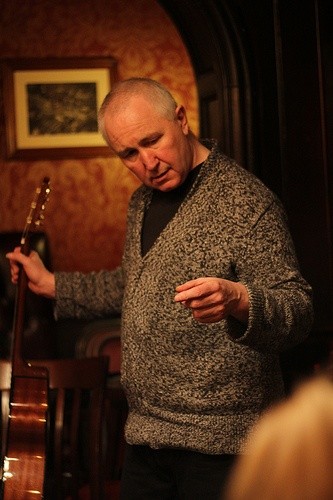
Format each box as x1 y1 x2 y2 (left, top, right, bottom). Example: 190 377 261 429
5 77 317 500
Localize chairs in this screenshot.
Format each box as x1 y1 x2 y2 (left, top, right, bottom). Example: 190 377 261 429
0 353 112 500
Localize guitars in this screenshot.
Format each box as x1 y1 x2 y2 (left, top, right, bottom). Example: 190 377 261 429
0 174 55 500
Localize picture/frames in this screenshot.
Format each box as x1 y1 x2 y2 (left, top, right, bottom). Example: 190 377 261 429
0 56 117 160
